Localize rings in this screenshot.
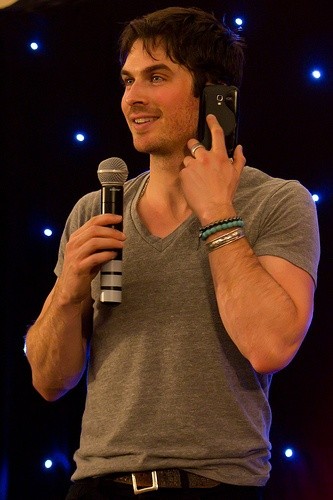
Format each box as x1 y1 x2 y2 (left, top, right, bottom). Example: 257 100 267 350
192 143 204 156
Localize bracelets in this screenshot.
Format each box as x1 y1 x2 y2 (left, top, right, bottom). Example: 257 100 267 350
204 228 245 253
200 216 242 230
202 221 244 239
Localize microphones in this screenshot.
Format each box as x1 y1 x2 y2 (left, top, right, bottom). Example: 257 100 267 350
97 157 129 304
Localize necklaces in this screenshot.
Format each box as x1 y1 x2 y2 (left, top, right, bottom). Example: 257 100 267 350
139 177 150 199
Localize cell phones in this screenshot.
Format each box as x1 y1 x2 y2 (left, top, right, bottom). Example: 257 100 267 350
202 85 240 158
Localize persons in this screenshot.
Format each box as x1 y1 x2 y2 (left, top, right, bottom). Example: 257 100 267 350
25 8 321 500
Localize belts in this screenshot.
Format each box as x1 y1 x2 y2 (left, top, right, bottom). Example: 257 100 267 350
86 469 221 495
